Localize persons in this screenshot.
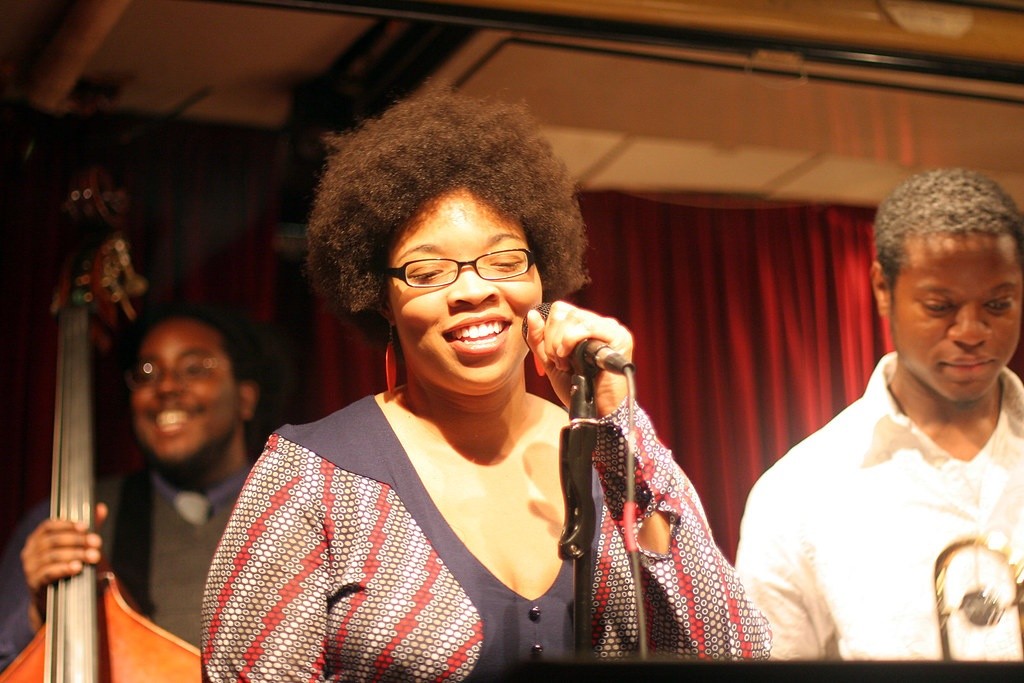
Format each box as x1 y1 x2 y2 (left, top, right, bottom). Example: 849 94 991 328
732 168 1024 663
0 310 266 675
199 89 774 683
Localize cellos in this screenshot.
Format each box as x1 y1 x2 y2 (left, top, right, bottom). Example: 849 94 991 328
0 160 203 683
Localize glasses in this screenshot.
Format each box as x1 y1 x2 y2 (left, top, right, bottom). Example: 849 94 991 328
384 248 538 288
123 353 228 390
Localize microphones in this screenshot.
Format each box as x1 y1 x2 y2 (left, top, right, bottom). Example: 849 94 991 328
522 302 635 376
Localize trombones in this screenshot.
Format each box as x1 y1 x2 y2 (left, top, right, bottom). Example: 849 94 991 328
933 530 1022 665
